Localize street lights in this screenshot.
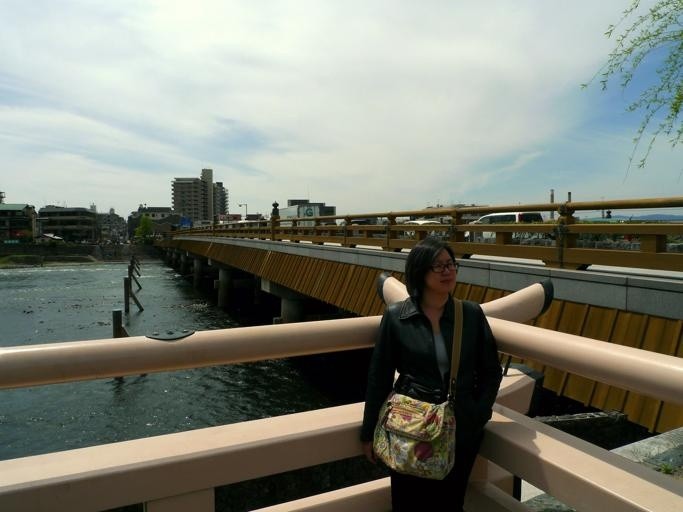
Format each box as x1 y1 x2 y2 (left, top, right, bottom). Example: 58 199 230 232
238 203 247 218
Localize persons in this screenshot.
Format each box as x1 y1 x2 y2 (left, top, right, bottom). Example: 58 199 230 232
358 237 503 511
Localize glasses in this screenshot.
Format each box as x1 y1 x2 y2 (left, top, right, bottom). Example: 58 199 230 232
430 262 459 273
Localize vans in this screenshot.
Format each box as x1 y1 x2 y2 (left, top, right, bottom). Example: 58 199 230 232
463 212 545 241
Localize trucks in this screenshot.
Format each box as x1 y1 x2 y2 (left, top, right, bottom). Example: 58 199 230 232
266 202 336 245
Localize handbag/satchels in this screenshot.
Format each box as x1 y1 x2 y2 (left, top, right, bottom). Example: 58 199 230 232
372 390 456 480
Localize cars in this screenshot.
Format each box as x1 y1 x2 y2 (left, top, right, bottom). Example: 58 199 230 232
316 219 449 240
